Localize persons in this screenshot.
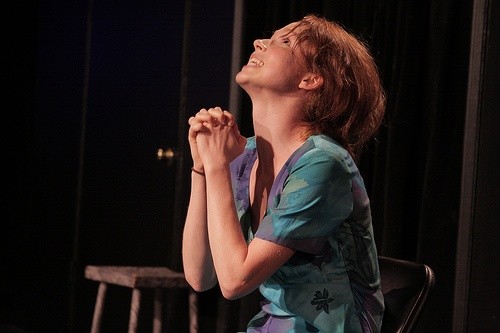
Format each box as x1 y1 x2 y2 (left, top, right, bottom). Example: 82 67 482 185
180 14 391 332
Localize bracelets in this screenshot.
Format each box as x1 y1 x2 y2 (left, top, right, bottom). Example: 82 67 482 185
190 166 207 177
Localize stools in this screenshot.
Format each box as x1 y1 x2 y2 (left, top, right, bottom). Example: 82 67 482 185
85 265 203 333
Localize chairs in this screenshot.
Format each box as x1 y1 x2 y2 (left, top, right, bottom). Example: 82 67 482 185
378 255 434 333
